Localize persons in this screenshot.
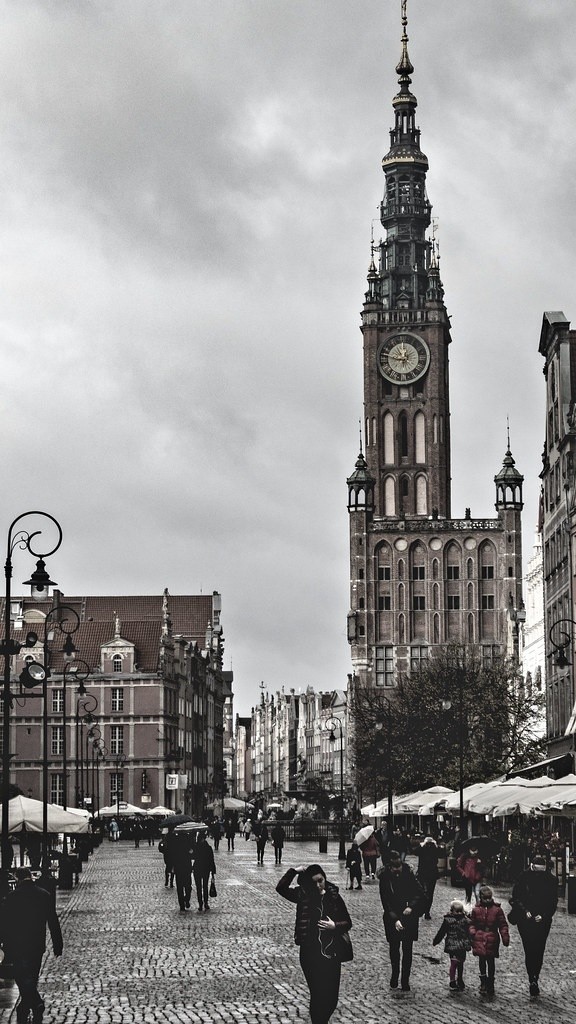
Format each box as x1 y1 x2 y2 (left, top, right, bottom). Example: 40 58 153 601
109 818 120 842
238 817 253 841
360 821 379 883
469 881 511 1003
271 822 286 867
508 855 559 997
252 818 269 867
346 844 363 890
431 900 472 991
456 841 486 902
275 864 353 1024
1 866 64 1024
132 817 157 848
379 849 424 991
417 837 439 920
158 816 235 912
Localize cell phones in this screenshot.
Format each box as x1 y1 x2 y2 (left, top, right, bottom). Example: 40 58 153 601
320 918 328 930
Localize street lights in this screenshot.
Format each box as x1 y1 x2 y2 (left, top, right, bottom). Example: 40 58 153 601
1 511 61 972
19 606 81 913
114 754 127 819
370 695 393 833
324 717 347 860
440 672 467 842
61 660 109 888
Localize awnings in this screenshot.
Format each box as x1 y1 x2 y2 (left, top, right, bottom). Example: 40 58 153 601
206 797 254 808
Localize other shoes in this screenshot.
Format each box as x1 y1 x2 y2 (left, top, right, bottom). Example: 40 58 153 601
180 904 185 911
457 977 465 988
197 907 202 911
449 980 458 991
355 884 362 890
366 875 370 879
15 1006 31 1023
204 904 210 910
32 1003 45 1024
529 982 540 997
401 978 410 991
345 886 353 890
372 873 375 879
425 913 431 919
390 974 399 988
184 896 190 908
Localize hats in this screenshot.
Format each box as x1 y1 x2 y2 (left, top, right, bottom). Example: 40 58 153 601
531 857 546 865
420 837 438 848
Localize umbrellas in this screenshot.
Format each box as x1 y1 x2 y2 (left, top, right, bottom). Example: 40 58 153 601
453 835 502 862
360 773 576 831
98 801 148 814
353 825 374 846
267 803 284 812
0 794 90 832
141 805 176 815
173 822 209 831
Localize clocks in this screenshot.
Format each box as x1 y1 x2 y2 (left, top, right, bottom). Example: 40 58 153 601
376 332 431 384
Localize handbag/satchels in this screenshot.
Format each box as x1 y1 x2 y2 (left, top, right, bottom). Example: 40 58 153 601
338 932 354 961
508 895 531 925
209 877 217 897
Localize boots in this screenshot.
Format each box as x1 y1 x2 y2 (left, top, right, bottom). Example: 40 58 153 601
487 977 495 995
479 975 487 993
